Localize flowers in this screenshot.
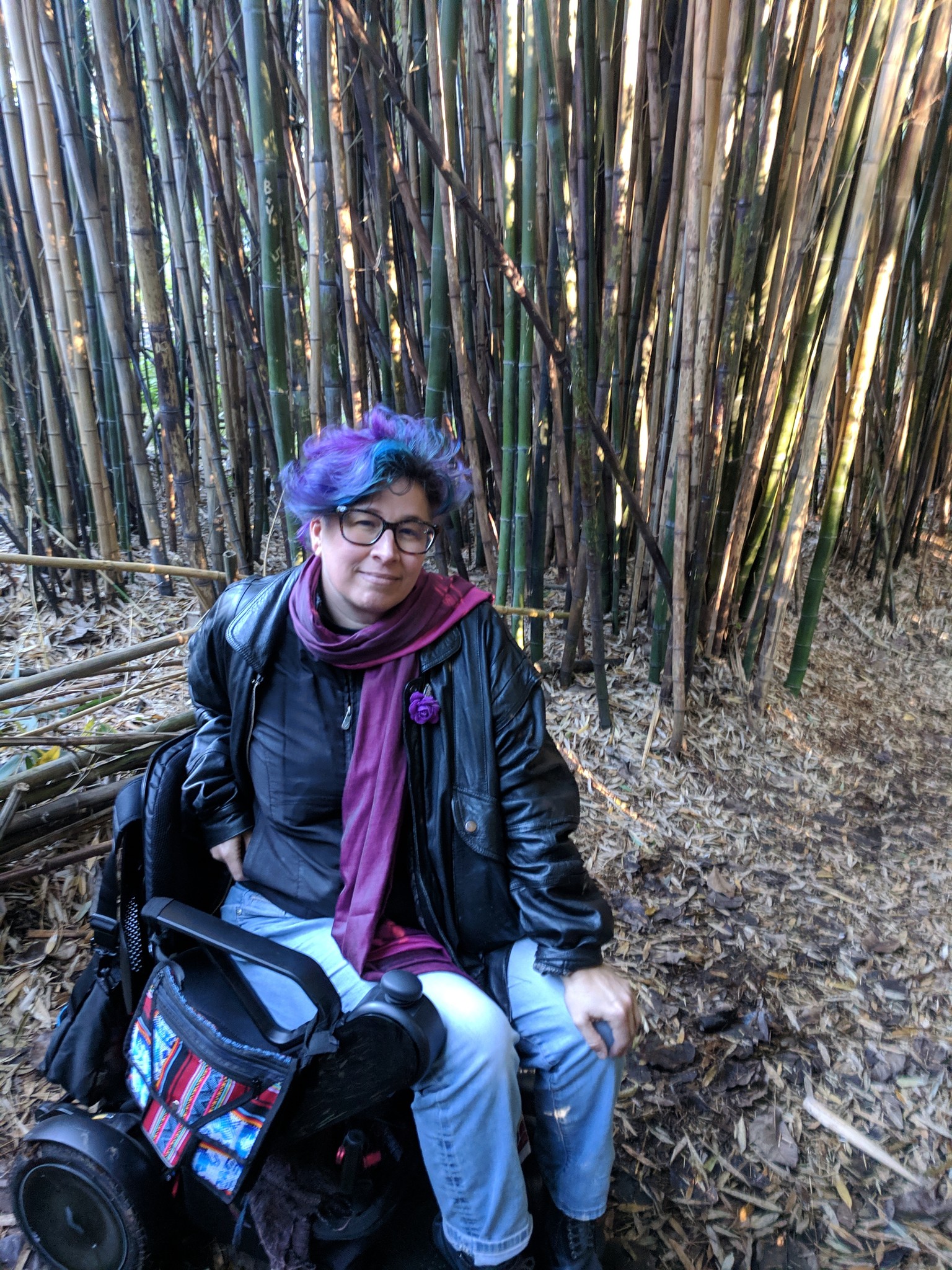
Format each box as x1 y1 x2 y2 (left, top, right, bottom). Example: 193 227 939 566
407 685 442 723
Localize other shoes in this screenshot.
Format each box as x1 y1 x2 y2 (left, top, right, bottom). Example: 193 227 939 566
544 1207 606 1270
431 1211 537 1270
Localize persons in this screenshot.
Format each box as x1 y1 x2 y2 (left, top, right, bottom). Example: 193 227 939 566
182 405 640 1270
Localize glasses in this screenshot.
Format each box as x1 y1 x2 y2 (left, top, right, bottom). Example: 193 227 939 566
335 506 442 554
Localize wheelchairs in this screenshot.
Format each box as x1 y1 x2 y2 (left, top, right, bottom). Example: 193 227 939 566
7 725 450 1270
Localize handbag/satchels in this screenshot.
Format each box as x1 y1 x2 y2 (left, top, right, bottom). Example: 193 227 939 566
39 855 131 1104
